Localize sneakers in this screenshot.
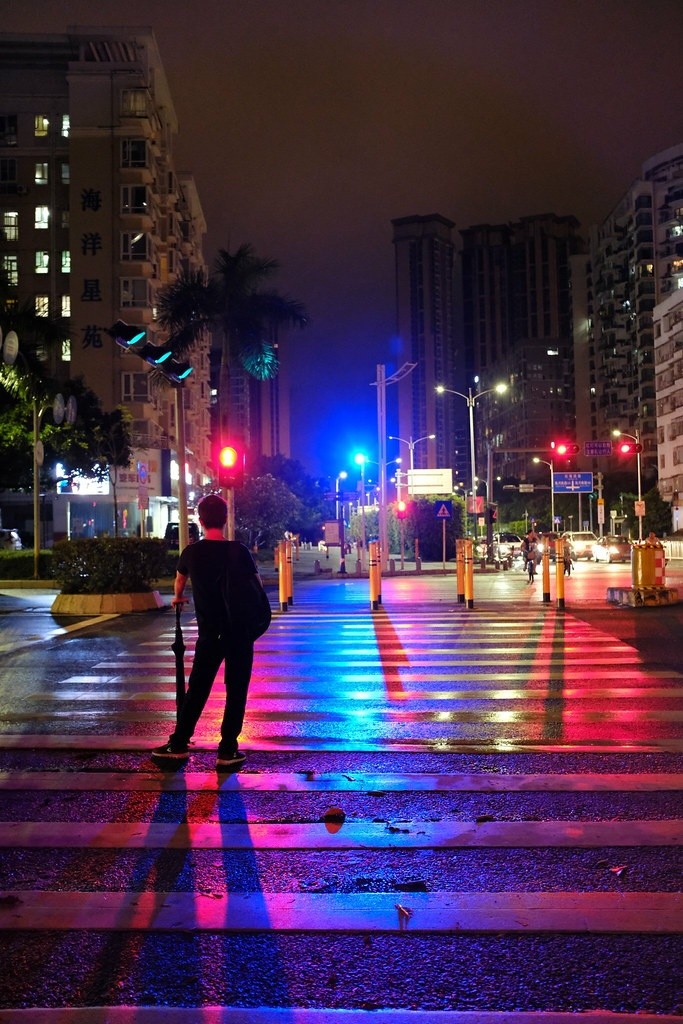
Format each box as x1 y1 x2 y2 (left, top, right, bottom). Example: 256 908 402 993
152 740 190 759
215 750 248 766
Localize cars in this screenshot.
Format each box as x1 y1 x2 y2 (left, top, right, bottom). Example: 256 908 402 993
521 533 559 563
560 531 597 561
488 533 524 562
0 527 23 553
592 535 634 564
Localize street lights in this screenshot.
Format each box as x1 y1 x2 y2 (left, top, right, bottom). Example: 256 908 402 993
434 384 508 541
534 457 555 533
336 470 348 520
388 433 435 468
612 428 643 544
347 452 501 544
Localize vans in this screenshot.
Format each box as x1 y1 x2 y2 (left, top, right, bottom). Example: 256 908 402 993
164 523 199 550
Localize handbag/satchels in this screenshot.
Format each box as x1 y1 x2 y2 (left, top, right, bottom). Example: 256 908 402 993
211 540 272 650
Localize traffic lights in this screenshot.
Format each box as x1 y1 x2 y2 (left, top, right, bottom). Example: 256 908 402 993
396 501 408 521
558 444 581 456
618 442 642 456
219 442 246 488
116 321 193 385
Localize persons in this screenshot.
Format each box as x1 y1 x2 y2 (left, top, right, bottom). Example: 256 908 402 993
521 530 574 574
151 495 264 764
645 530 662 544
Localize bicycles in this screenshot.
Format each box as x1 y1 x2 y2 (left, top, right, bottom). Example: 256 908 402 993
564 546 573 575
521 547 540 583
